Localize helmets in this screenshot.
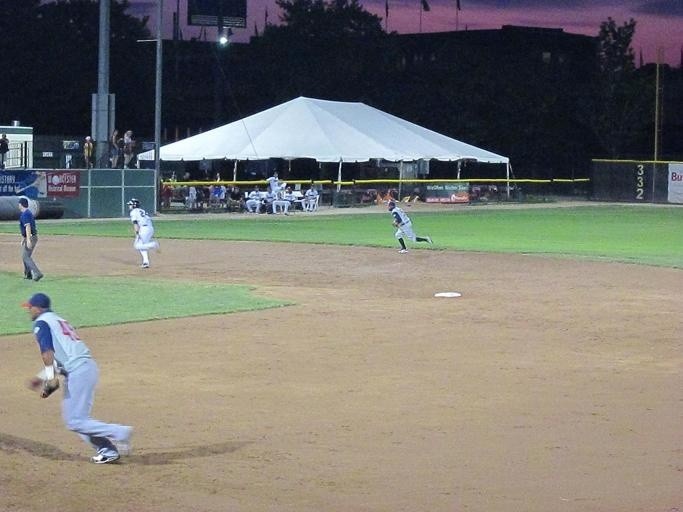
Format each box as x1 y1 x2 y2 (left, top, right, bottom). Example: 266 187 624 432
125 199 139 208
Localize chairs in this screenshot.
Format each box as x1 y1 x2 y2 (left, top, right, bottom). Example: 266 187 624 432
185 185 320 216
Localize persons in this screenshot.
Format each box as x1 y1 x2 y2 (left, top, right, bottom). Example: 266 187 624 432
123 129 134 169
83 136 94 169
110 128 123 169
0 131 10 169
126 198 160 270
17 198 44 282
18 292 134 464
387 201 434 254
162 170 318 215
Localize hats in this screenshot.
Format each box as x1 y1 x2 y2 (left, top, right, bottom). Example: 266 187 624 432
385 202 395 207
17 198 28 208
19 293 49 308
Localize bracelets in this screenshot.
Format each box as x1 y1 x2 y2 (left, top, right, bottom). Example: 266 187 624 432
45 364 54 381
36 370 48 381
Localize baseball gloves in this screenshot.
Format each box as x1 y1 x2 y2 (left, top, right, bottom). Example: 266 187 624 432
39 379 59 398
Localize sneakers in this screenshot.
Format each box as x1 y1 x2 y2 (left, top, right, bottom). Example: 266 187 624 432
88 449 119 464
426 236 433 245
138 262 149 268
397 249 409 254
116 425 133 457
21 272 43 281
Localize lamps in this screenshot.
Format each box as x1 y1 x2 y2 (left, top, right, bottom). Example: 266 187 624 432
219 27 231 46
